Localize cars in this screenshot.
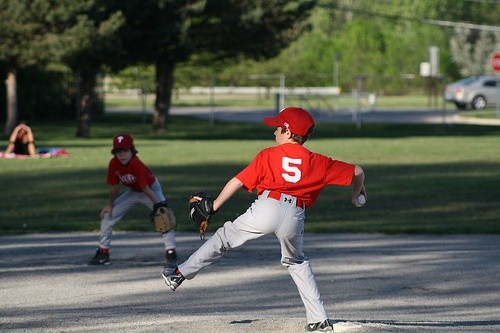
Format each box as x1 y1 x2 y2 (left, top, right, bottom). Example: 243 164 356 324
444 74 500 111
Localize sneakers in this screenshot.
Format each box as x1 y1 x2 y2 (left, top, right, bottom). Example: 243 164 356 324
161 266 186 291
305 318 334 333
165 248 177 262
88 247 110 264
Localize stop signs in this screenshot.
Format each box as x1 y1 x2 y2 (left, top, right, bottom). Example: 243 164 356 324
491 53 500 71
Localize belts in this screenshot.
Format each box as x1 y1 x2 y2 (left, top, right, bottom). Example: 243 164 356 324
267 191 303 209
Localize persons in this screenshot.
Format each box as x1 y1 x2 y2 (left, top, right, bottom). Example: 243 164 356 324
5 122 35 155
88 133 177 275
161 107 367 333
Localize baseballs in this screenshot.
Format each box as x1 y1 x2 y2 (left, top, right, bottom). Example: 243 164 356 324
356 194 366 205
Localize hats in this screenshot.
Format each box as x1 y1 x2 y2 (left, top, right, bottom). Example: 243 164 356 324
113 135 133 149
264 106 315 136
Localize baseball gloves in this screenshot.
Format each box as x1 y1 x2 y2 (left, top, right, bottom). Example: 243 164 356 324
189 191 215 235
150 205 178 233
